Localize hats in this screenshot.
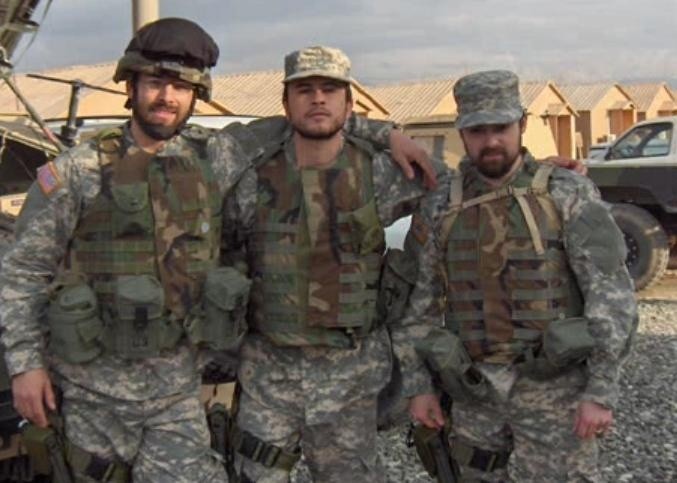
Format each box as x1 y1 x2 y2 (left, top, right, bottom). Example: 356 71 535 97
453 69 523 130
280 45 353 84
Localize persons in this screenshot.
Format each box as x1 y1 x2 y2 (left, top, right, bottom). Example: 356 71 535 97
390 70 639 483
220 46 587 483
1 19 441 483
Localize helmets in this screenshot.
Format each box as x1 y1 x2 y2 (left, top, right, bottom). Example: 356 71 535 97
112 35 212 101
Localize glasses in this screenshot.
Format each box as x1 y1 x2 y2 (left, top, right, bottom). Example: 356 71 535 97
124 17 219 67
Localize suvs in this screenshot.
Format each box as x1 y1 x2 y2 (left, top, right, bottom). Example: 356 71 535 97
580 115 677 293
